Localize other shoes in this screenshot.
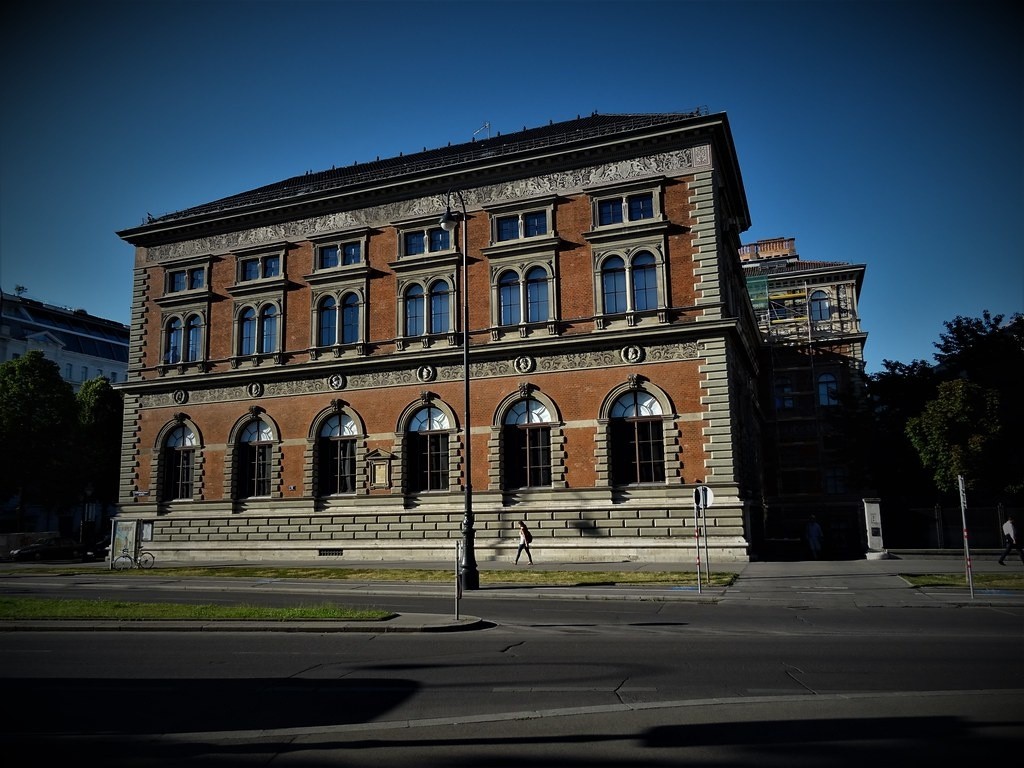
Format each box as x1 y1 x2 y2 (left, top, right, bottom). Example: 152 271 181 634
999 560 1006 566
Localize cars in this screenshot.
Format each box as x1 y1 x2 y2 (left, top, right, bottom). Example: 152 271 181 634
75 536 110 562
8 536 78 561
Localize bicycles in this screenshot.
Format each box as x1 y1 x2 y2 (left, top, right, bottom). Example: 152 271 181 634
113 546 154 569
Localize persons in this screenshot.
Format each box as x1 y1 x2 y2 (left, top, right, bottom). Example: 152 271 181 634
510 521 533 565
20 534 33 544
999 516 1024 566
806 515 823 559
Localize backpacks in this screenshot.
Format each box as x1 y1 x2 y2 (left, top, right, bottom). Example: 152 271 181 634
522 527 533 544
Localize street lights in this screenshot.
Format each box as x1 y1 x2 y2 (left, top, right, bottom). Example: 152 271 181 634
439 185 481 590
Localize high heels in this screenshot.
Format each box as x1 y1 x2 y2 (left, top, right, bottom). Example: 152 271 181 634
528 562 533 565
515 563 517 565
